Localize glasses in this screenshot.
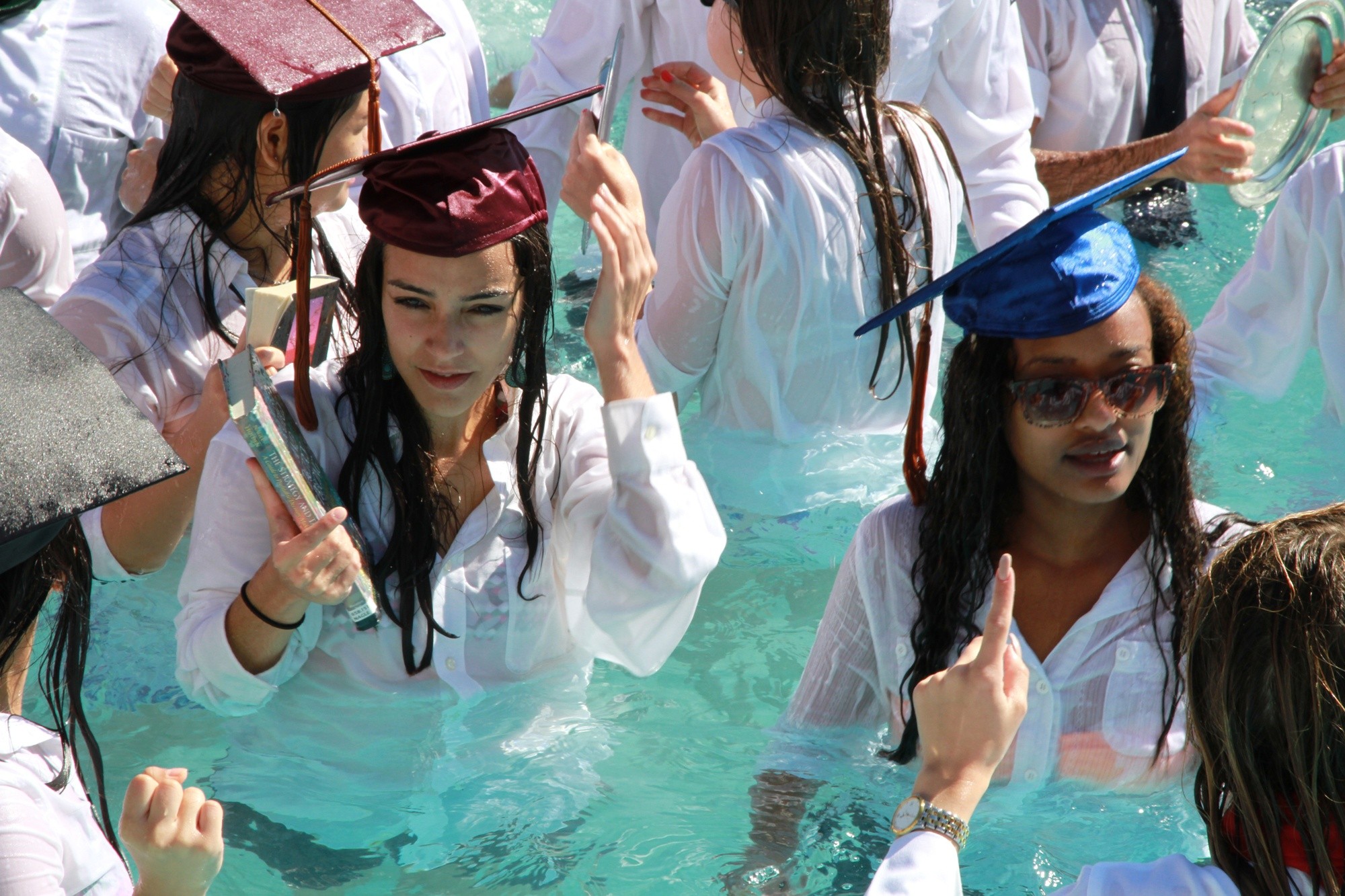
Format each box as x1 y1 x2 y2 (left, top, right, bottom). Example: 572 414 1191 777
1001 361 1176 429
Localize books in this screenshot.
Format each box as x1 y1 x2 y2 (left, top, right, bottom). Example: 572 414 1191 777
245 274 341 369
218 344 383 631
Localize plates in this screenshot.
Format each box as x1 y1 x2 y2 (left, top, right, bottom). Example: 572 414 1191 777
1225 0 1345 208
581 22 625 255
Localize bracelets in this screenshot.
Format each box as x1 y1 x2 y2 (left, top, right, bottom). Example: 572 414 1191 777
241 581 306 630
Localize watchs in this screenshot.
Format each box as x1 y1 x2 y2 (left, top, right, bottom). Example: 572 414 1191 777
891 796 969 851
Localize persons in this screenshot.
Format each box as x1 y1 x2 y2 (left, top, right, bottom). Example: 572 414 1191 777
864 501 1345 896
175 87 728 701
788 147 1251 785
0 287 226 896
1 0 490 310
49 0 447 579
508 0 1047 273
562 0 976 431
1189 141 1344 435
1017 0 1345 204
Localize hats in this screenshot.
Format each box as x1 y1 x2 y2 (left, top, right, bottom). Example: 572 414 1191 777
1 288 191 569
856 146 1190 502
266 86 604 432
173 0 446 154
